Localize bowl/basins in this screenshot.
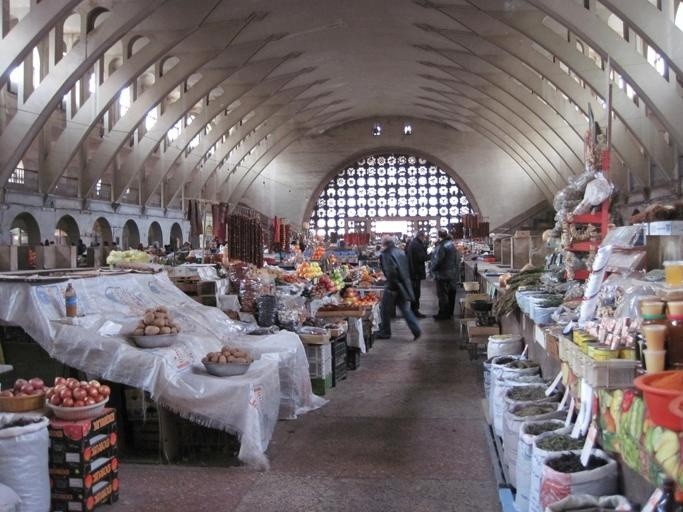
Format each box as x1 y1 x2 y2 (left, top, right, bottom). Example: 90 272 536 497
127 332 178 348
631 370 683 432
470 300 492 310
200 359 252 377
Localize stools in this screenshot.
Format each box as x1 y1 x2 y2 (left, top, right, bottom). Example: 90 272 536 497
467 321 501 360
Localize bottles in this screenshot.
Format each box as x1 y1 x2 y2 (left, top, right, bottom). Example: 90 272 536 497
64 283 77 316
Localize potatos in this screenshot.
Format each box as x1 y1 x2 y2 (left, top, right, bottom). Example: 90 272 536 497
203 345 251 364
134 306 181 336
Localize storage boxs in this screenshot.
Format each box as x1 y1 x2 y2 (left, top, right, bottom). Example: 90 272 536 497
46 405 120 512
123 387 158 452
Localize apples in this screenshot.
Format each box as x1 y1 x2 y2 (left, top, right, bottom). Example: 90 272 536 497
0 377 111 408
294 262 335 291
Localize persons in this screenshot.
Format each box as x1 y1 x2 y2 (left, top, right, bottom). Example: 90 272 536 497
376 228 462 341
70 238 306 265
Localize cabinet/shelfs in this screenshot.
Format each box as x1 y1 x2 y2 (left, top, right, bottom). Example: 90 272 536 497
558 150 609 283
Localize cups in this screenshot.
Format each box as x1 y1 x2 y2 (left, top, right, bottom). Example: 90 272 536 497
643 325 668 352
195 258 201 264
640 349 667 370
662 260 682 289
572 328 637 361
641 299 664 319
665 301 683 320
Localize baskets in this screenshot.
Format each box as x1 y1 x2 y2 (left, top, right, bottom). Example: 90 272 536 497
0 391 46 413
303 342 347 395
45 395 109 421
